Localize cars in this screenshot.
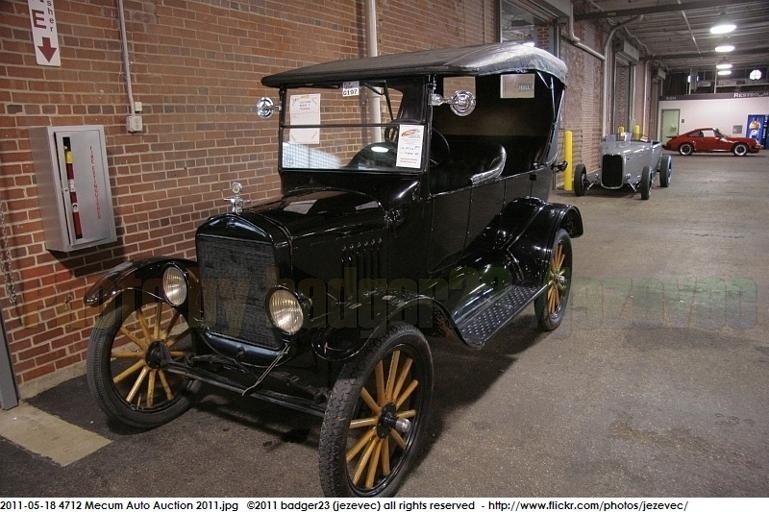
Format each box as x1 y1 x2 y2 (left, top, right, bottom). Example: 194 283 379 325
572 130 672 200
81 37 583 497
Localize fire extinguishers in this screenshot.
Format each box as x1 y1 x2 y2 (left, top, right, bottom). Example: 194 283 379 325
62 137 82 235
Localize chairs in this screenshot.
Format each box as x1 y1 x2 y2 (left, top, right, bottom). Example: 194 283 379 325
427 135 546 188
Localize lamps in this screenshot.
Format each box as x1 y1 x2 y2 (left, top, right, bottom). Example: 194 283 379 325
716 57 732 69
710 13 737 34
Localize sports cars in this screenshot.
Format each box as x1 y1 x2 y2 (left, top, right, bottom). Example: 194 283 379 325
667 127 762 157
